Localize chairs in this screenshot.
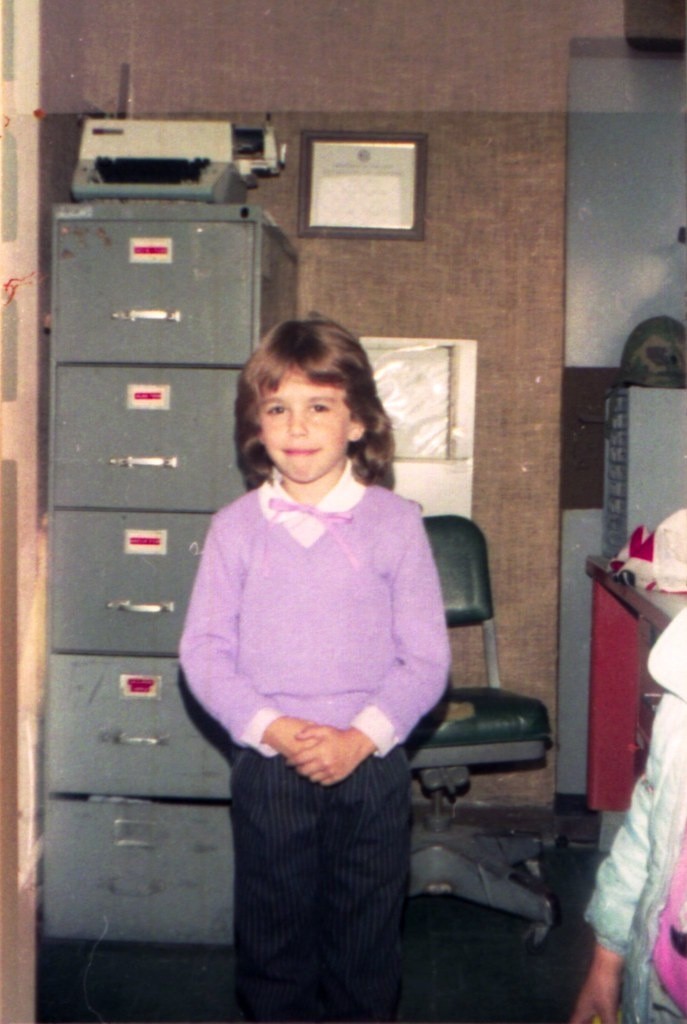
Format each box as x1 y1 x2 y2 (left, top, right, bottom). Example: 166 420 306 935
397 514 565 952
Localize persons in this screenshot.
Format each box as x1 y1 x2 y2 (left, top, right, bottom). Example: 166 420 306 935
571 610 687 1024
179 320 450 1024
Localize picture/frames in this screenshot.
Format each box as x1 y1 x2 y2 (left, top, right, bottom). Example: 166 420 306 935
296 130 429 242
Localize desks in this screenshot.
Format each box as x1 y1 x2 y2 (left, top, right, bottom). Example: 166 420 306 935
580 552 687 857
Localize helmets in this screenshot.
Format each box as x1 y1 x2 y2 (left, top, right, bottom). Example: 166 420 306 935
608 316 685 385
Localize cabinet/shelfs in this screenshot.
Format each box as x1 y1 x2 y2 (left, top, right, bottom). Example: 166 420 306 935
41 200 299 950
600 384 687 560
